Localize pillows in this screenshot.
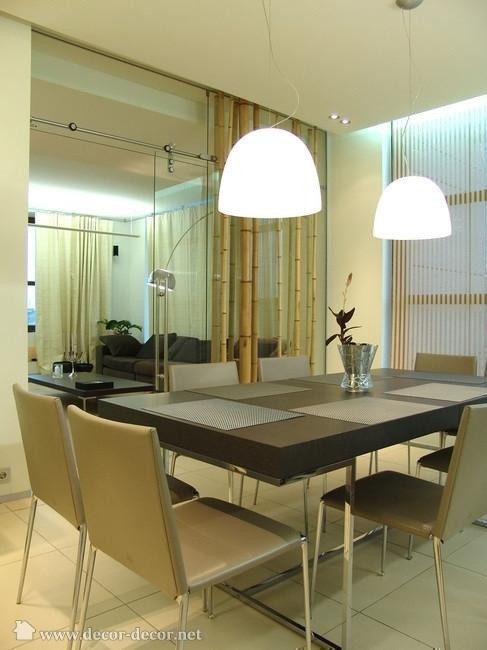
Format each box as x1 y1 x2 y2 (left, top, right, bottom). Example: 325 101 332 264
99 335 141 356
137 332 176 360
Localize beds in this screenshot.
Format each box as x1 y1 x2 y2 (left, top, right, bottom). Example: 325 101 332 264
28 371 155 413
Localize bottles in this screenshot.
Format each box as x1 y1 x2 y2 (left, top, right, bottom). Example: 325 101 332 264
55 365 60 373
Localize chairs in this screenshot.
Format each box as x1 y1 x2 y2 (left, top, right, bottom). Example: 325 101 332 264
12 382 209 650
66 403 312 650
253 356 312 506
309 402 487 650
369 351 479 485
168 360 245 507
407 445 487 560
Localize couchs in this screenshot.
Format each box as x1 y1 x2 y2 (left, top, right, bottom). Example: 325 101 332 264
95 337 211 384
227 335 292 363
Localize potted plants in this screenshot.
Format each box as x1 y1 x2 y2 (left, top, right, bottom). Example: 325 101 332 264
323 271 379 395
96 319 142 336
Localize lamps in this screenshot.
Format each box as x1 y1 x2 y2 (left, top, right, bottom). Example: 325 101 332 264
217 0 322 219
372 0 453 241
145 210 214 294
